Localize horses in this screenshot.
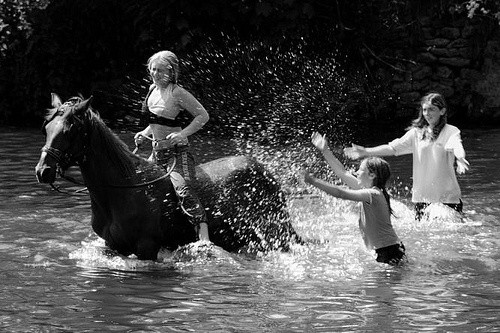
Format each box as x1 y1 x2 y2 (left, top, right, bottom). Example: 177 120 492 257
34 91 307 262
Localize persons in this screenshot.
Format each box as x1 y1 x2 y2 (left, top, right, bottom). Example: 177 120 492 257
342 93 471 221
290 131 408 268
134 50 210 242
